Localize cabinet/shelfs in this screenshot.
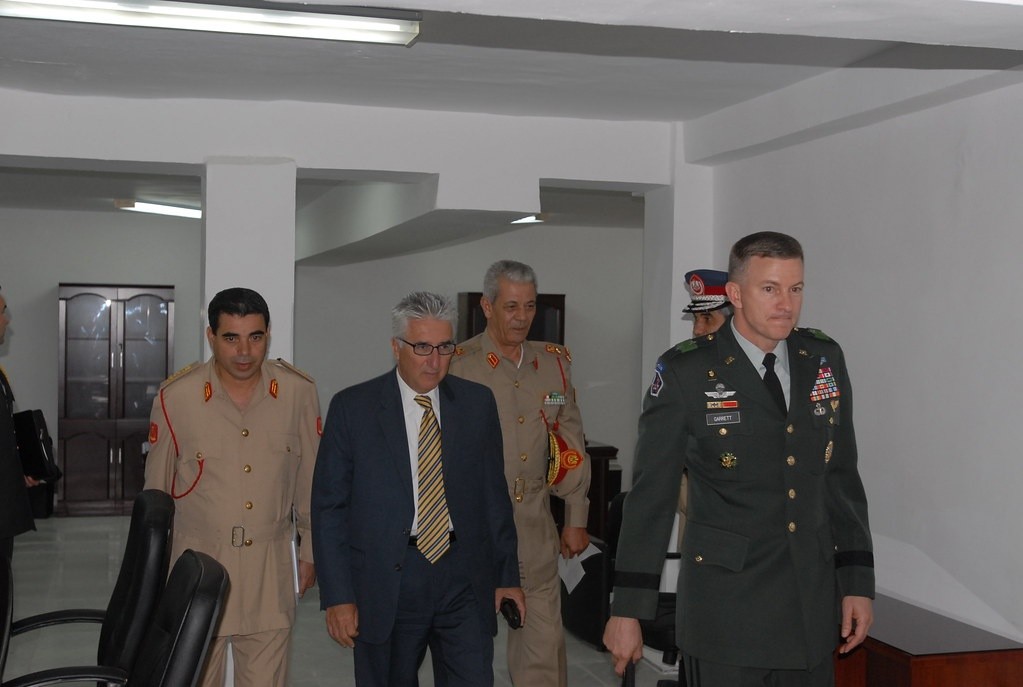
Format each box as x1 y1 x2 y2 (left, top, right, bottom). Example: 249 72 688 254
559 437 630 651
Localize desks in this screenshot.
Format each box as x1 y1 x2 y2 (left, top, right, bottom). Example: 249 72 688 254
835 590 1023 687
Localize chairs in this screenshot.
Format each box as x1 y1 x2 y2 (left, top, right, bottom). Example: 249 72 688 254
0 548 233 687
5 490 175 687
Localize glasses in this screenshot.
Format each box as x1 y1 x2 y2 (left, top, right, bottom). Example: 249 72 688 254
397 337 457 356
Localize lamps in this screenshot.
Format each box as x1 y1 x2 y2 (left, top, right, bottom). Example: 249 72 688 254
112 199 204 219
1 0 427 50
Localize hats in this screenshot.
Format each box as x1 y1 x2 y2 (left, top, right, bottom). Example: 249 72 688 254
539 422 586 500
681 268 733 313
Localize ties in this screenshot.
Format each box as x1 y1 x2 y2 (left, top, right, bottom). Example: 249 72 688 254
413 395 450 564
761 353 787 417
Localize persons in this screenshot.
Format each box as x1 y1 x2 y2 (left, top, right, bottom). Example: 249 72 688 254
449 257 591 686
140 289 322 687
673 268 734 557
308 293 528 687
0 286 36 644
600 233 875 686
